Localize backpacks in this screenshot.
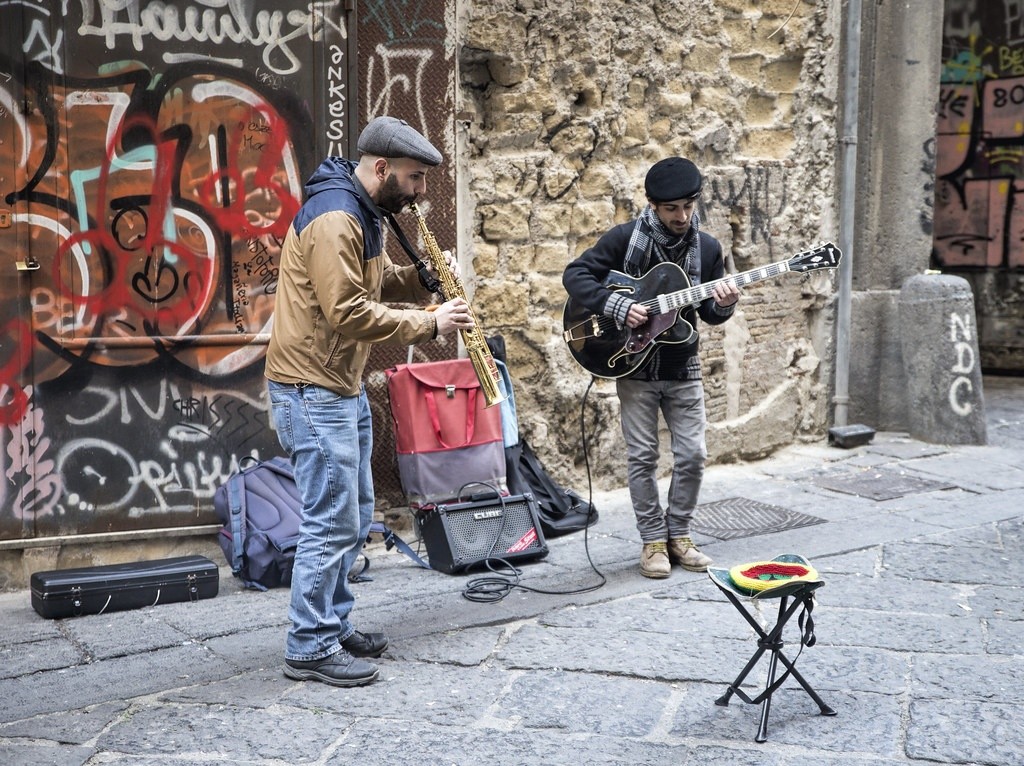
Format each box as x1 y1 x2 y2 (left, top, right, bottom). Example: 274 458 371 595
505 430 599 539
214 456 384 592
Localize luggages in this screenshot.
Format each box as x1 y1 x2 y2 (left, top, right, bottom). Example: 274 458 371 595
385 358 511 506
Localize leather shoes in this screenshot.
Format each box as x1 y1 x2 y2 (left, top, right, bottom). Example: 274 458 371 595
339 630 389 657
283 650 379 688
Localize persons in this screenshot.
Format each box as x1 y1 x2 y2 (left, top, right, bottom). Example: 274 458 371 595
563 156 740 580
264 117 477 688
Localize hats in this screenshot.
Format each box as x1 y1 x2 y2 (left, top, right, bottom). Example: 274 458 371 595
358 117 443 167
645 157 703 205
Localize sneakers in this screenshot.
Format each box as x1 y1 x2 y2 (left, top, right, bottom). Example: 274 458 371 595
670 538 714 573
639 542 671 579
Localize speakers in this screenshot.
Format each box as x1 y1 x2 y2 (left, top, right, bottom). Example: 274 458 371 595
420 492 550 576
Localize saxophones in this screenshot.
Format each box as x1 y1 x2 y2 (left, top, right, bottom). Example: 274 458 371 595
407 199 511 410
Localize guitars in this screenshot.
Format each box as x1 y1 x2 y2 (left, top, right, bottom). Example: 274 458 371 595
562 242 842 380
